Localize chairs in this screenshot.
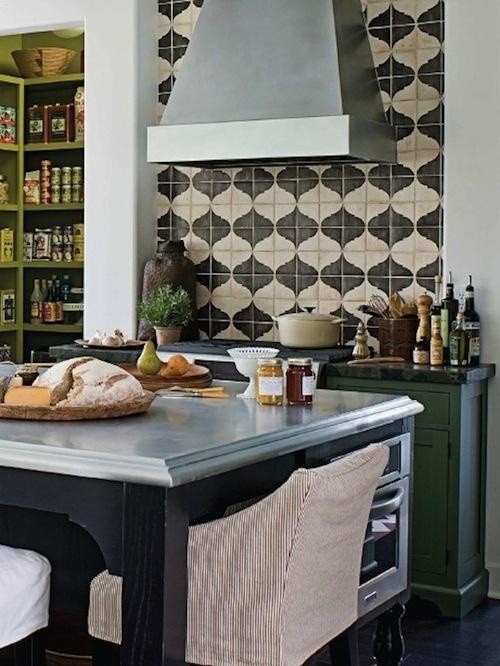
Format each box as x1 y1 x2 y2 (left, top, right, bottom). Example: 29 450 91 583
0 545 52 666
86 442 391 666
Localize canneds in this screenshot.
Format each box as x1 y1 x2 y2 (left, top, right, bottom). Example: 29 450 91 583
286 358 314 407
40 159 82 204
51 223 85 263
256 358 284 405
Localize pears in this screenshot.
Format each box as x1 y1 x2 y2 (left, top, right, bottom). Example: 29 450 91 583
136 339 162 375
161 354 190 377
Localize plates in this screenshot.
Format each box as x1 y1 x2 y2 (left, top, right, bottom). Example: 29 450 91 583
117 360 217 389
75 337 147 348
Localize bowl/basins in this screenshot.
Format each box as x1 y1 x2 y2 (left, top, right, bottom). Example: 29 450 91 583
5 48 78 76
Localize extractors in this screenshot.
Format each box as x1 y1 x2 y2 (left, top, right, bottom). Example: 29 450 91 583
146 0 398 168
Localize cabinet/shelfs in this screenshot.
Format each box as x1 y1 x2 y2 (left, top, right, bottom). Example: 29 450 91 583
0 75 25 365
323 376 490 619
24 73 85 363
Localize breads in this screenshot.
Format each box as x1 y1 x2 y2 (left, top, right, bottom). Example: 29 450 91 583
32 355 143 409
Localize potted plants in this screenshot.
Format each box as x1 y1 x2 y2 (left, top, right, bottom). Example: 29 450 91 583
134 282 195 346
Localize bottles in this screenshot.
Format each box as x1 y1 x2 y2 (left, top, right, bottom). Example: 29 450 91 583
411 274 482 370
31 275 64 325
352 322 371 362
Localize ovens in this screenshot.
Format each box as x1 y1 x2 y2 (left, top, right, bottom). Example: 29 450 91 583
305 421 413 626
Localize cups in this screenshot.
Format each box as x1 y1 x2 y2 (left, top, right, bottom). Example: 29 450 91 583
376 318 409 357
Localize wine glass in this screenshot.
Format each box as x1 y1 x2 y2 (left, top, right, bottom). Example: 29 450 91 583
229 347 279 400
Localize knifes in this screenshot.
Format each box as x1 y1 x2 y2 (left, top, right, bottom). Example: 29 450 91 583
152 388 230 401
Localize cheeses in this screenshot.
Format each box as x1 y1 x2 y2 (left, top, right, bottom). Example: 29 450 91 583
4 384 50 408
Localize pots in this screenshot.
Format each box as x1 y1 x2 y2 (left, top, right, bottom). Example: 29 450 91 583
268 307 345 349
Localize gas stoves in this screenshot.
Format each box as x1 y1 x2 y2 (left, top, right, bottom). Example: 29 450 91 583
154 338 358 391
